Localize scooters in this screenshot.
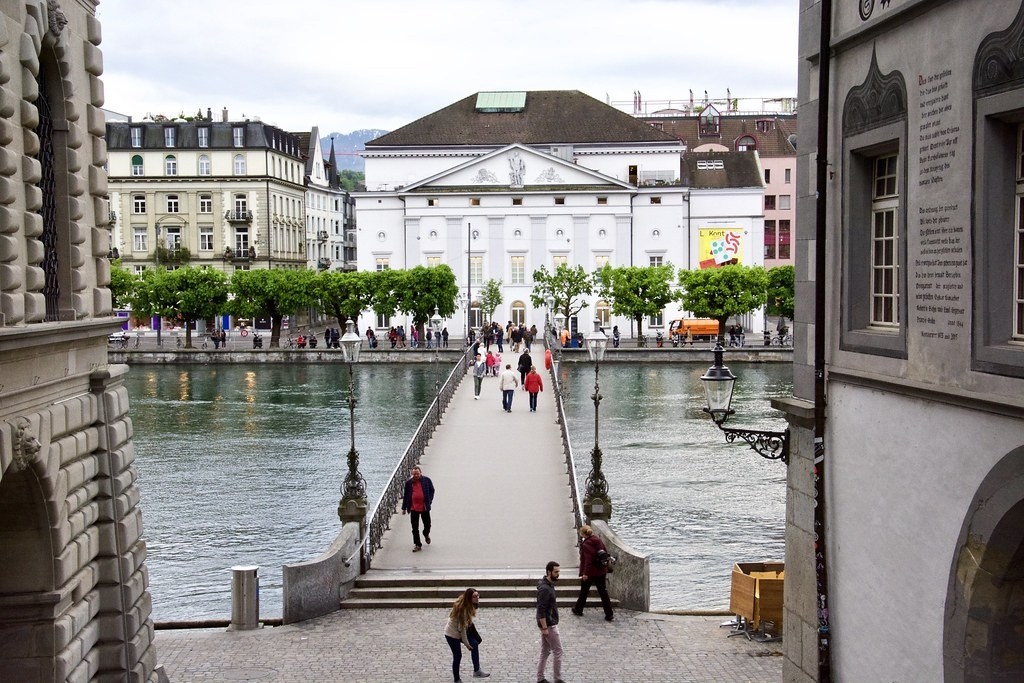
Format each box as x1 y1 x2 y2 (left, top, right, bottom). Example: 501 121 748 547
612 331 621 348
654 328 687 348
284 330 317 349
252 331 263 349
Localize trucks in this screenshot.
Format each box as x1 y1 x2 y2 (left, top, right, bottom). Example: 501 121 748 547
669 318 720 340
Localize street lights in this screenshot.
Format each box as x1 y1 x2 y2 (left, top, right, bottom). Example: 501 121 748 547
337 314 371 532
584 315 613 524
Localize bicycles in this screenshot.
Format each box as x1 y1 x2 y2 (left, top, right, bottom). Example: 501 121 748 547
116 334 130 349
132 334 142 349
772 334 793 347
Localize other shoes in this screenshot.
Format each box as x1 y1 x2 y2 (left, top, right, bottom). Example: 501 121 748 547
605 616 613 621
555 679 565 683
412 546 421 552
473 667 490 677
537 679 549 683
474 395 479 399
424 534 431 544
572 608 583 616
456 679 463 683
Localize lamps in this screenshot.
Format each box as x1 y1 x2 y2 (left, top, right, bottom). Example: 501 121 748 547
700 337 790 466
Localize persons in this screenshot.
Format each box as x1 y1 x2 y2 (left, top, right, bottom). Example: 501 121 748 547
426 327 449 349
672 327 693 347
473 340 501 399
366 328 374 348
728 324 742 346
481 322 503 353
298 328 340 349
537 561 565 683
389 325 418 348
613 327 619 348
506 320 538 353
211 330 226 349
445 588 490 683
552 327 583 348
500 364 518 412
779 326 786 347
525 367 543 412
572 525 613 620
517 348 532 389
402 467 435 552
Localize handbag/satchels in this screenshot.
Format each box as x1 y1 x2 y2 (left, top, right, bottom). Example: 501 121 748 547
517 364 523 372
466 623 482 645
593 550 608 569
